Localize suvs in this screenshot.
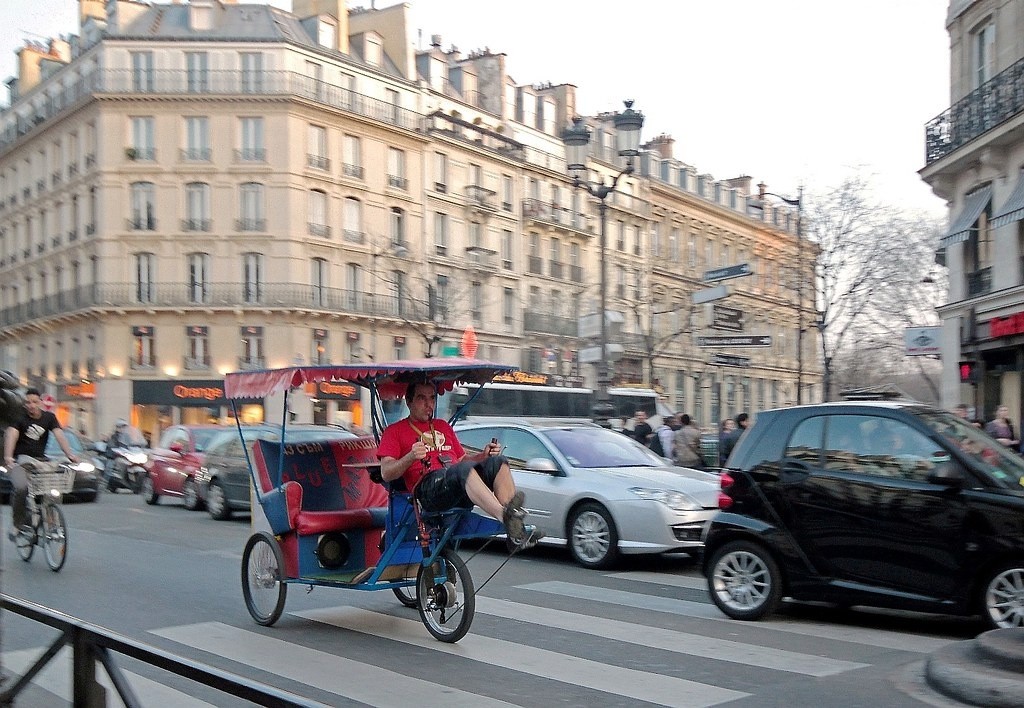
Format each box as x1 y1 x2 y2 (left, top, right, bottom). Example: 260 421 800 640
196 418 360 520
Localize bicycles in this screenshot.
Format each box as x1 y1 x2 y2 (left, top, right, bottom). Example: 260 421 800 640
0 455 86 572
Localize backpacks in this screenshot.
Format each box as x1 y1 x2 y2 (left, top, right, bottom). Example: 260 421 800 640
650 427 669 458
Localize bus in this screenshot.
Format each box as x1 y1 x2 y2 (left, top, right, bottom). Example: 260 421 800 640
399 382 661 429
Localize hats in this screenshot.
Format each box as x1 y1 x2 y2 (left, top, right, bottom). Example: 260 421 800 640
115 418 127 427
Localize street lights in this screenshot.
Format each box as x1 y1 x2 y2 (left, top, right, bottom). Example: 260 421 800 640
748 192 803 407
560 101 643 428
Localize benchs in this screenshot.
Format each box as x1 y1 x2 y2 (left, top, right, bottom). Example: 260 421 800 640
251 436 413 535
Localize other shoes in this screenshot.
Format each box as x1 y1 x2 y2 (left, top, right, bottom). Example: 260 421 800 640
15 522 30 533
506 491 526 539
506 530 543 554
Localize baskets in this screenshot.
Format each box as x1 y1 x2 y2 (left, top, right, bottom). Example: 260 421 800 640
27 471 76 495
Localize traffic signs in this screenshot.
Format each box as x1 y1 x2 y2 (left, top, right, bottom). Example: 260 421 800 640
696 336 773 347
701 263 752 282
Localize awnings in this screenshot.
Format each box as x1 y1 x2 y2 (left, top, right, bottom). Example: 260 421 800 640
939 186 992 249
989 175 1024 229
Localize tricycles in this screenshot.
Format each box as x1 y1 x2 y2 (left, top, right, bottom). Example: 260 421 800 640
223 358 546 643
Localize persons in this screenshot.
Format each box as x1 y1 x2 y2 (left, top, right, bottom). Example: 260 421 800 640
104 418 141 489
622 409 748 473
951 405 1020 454
3 388 79 542
378 378 544 549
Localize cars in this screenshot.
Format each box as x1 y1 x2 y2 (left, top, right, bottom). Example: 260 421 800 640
142 424 223 511
0 428 100 505
451 420 722 571
701 399 1024 628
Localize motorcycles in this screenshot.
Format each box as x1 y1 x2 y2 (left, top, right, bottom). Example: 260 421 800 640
96 425 149 494
